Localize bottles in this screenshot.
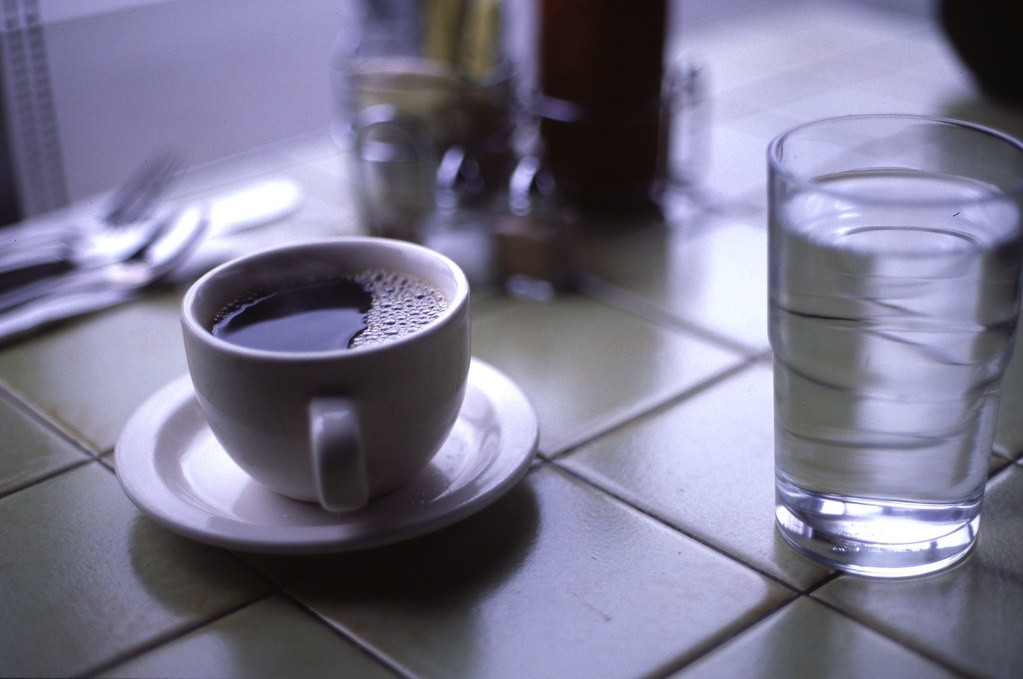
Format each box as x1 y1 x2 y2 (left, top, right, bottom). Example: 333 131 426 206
356 2 510 232
535 0 671 227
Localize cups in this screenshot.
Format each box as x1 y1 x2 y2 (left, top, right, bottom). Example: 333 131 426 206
334 60 518 288
180 234 471 512
767 116 1023 580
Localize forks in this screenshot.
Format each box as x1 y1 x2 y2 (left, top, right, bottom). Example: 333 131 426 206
0 146 184 271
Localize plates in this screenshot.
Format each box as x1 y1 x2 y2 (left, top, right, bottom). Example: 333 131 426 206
115 358 539 555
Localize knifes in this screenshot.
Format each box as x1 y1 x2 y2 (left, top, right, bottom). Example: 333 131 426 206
0 180 301 295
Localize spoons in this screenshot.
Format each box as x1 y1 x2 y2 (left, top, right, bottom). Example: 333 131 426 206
0 208 204 336
